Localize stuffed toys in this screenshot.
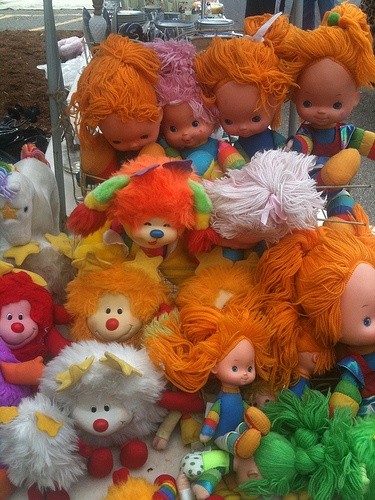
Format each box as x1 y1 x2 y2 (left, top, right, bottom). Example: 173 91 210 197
0 1 375 499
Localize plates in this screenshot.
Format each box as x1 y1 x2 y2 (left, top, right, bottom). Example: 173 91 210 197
117 6 235 39
174 32 265 43
